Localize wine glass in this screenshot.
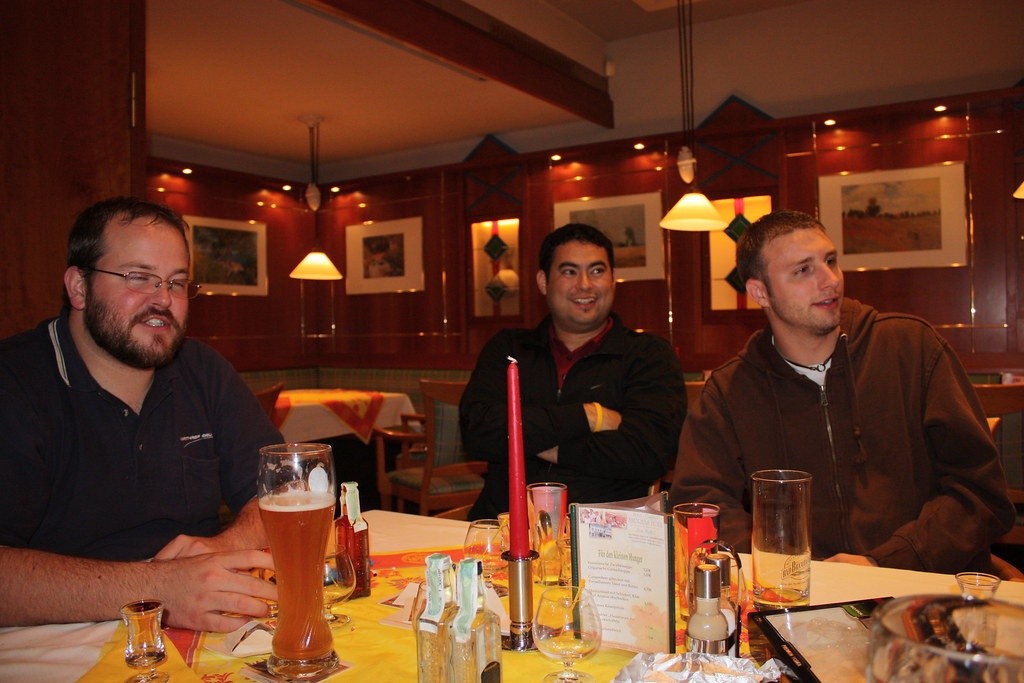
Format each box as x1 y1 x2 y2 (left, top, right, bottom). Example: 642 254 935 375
120 598 168 683
320 544 356 629
532 586 602 683
464 519 508 597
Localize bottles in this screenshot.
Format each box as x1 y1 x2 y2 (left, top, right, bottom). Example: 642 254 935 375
334 480 371 598
417 551 502 683
685 540 743 658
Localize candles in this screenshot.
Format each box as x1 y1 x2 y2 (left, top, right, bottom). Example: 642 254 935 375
507 354 531 558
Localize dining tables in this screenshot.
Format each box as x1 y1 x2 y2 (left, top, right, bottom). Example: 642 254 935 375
0 507 1024 683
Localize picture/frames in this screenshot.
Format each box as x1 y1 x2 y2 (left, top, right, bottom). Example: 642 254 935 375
818 160 969 273
553 192 667 284
182 215 269 297
346 216 425 296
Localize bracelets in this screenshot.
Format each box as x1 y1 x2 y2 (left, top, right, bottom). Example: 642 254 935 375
592 402 602 432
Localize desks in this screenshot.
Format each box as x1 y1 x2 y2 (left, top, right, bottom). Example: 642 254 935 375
271 389 419 443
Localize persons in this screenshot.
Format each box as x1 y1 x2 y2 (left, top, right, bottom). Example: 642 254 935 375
0 198 302 634
669 211 1016 573
458 222 687 528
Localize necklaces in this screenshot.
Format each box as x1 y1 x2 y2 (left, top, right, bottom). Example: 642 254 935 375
785 353 833 372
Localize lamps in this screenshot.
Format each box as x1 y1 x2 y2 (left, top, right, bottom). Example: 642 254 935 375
288 113 344 280
658 0 729 232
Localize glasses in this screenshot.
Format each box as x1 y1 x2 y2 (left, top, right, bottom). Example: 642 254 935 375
70 262 203 299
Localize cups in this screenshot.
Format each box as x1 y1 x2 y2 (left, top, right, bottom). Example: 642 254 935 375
249 545 277 628
497 482 572 587
256 442 340 681
673 501 720 622
410 581 426 636
864 594 1023 682
751 469 811 611
955 571 1001 600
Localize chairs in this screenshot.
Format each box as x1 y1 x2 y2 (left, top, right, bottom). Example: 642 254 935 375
254 382 284 422
373 377 488 515
